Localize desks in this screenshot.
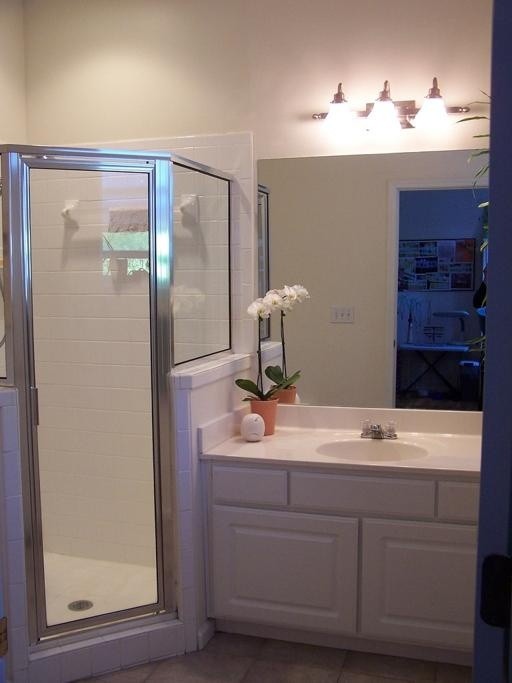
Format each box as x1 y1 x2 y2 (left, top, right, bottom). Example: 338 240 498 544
398 340 471 404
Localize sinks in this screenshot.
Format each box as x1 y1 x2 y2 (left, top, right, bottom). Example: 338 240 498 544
316 440 430 462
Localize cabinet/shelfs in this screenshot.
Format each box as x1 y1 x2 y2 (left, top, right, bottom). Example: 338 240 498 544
200 460 481 669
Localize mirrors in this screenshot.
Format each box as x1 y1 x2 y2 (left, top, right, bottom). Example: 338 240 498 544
256 149 489 413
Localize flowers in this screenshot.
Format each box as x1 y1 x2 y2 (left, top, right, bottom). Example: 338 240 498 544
231 293 281 403
266 284 310 390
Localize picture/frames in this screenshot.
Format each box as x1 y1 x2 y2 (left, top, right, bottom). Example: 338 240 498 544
398 238 477 292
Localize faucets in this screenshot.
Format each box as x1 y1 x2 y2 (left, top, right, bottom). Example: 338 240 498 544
369 423 384 439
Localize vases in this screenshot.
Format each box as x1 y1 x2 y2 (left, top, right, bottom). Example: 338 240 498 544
270 385 296 404
249 398 280 436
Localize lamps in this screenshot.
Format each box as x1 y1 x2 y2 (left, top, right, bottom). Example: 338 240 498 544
433 310 471 346
313 76 473 147
